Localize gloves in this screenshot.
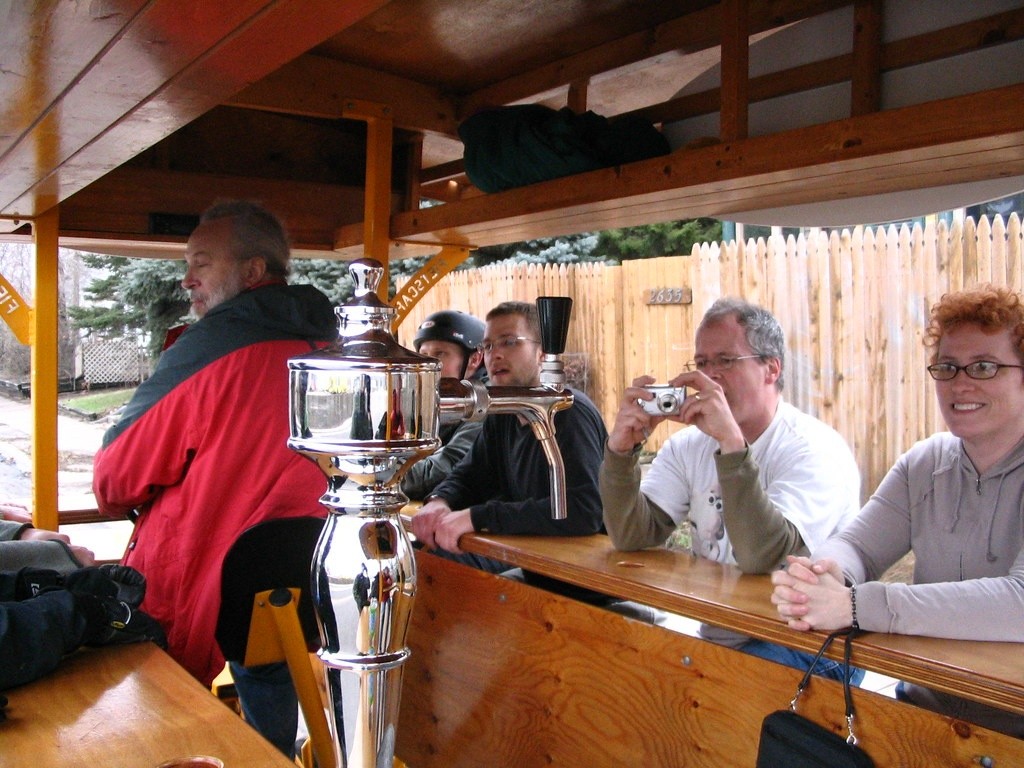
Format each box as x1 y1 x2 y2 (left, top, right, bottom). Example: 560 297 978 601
82 595 167 652
11 564 119 603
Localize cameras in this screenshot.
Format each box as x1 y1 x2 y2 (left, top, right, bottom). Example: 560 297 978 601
637 384 686 416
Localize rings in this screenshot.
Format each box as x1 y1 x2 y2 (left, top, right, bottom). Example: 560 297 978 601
695 392 702 401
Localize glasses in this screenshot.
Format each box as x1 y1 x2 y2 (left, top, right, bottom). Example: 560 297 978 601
683 353 763 372
927 363 1024 380
482 335 541 353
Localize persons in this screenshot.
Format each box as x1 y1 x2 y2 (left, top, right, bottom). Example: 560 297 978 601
0 520 169 710
399 300 629 608
771 283 1023 742
353 562 393 656
598 294 866 688
92 196 339 763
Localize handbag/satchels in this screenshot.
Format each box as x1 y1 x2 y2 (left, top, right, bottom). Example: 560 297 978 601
756 628 874 768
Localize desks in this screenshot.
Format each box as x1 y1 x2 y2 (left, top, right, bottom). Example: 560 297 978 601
400 499 1024 715
0 643 298 768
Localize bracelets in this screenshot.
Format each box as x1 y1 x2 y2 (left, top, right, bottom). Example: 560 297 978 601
427 494 438 503
850 585 858 630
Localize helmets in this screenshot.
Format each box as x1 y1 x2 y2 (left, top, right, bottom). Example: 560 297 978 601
413 310 486 351
